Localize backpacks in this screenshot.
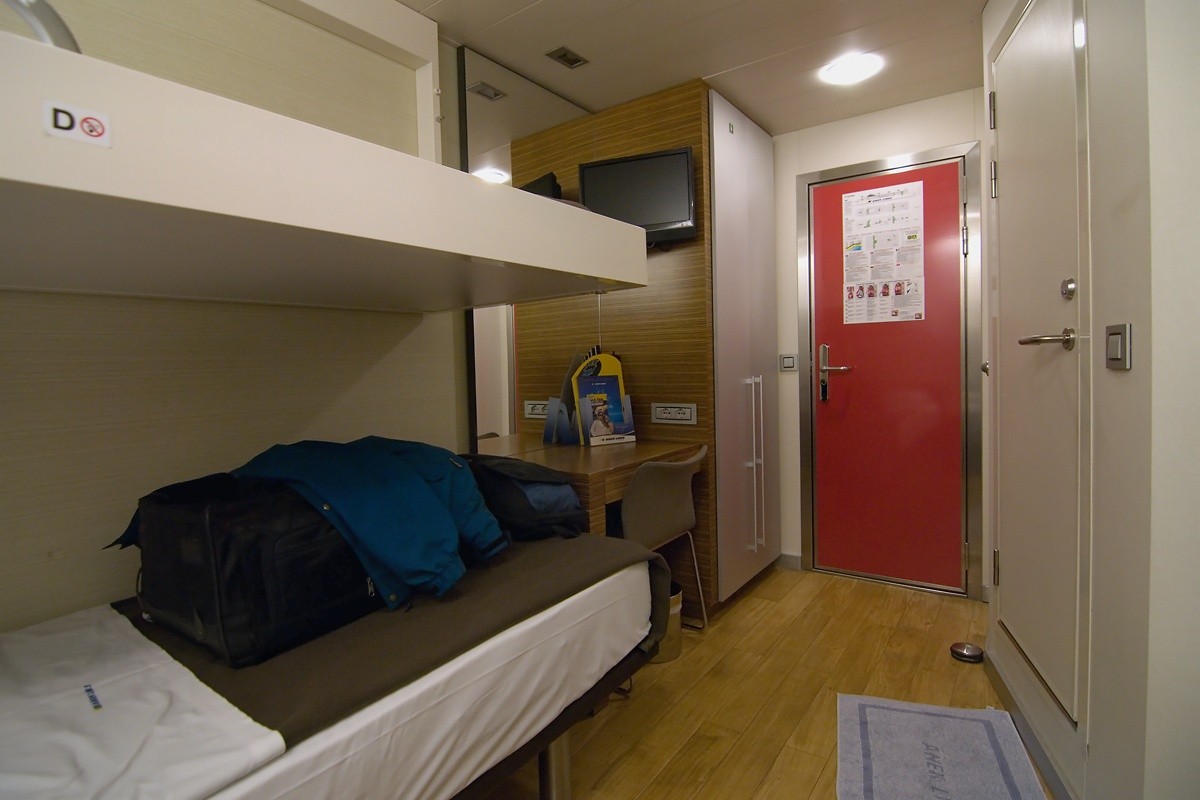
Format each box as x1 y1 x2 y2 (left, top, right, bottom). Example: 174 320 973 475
458 453 586 543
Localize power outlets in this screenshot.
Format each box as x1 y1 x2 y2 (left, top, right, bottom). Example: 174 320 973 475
651 402 698 423
524 400 549 419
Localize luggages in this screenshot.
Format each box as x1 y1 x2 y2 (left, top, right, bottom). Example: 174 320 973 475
138 471 471 669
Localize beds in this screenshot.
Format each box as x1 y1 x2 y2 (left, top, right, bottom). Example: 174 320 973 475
1 532 673 799
0 0 649 314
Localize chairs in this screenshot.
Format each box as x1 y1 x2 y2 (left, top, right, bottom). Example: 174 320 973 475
604 445 710 630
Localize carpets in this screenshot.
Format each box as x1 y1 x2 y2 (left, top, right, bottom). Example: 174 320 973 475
835 691 1047 800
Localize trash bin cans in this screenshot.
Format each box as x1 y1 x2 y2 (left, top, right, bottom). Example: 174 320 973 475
634 581 683 663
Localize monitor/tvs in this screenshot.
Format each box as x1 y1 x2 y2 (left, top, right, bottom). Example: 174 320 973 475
578 146 696 248
517 172 562 200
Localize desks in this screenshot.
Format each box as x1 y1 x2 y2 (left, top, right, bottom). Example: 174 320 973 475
474 433 707 534
